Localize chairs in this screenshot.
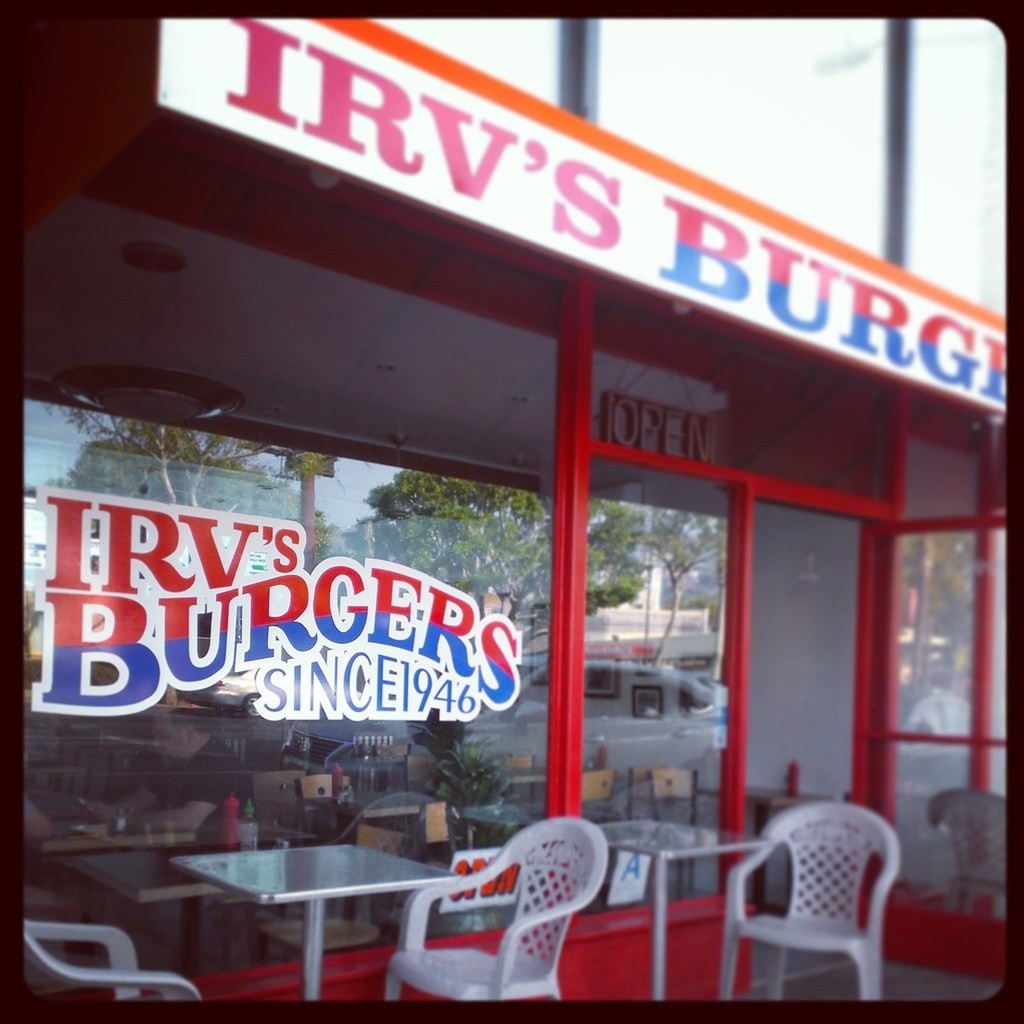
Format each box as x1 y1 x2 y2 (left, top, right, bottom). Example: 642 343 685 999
627 764 669 816
646 766 698 892
927 787 1005 915
582 766 618 824
376 817 609 1001
415 797 465 843
327 789 441 860
23 919 202 1000
403 753 440 791
254 822 427 968
292 773 357 848
717 800 903 1000
503 753 540 799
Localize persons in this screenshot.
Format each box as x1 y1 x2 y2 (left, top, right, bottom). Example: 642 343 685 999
76 708 256 856
23 799 81 925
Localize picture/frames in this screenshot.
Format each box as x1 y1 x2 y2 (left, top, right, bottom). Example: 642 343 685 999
631 683 664 720
584 590 624 699
530 603 549 686
676 654 714 717
479 592 517 623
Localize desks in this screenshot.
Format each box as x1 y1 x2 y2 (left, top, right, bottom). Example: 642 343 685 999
36 828 198 923
596 819 774 1000
699 784 836 918
507 766 547 783
298 793 431 845
168 844 462 1001
65 848 221 905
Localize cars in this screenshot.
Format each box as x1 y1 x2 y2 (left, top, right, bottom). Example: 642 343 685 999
173 653 722 831
